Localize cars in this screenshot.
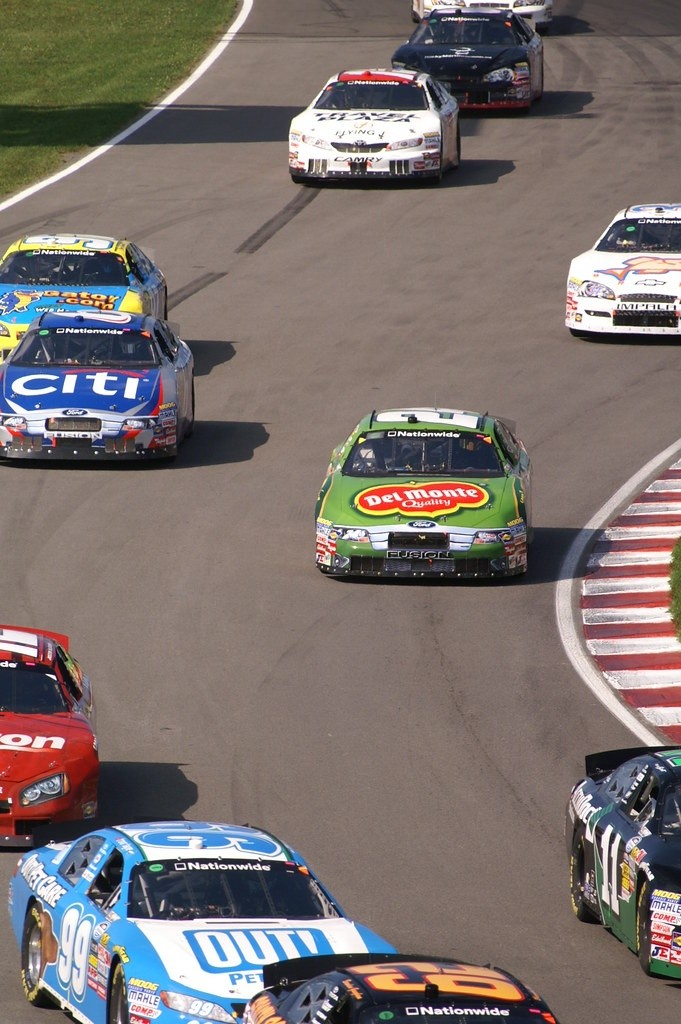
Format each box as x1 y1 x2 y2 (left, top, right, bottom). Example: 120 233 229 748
564 203 681 339
243 950 561 1024
9 805 400 1023
563 742 681 986
391 8 545 111
314 407 533 578
0 623 102 853
0 305 200 469
0 231 170 370
289 69 460 185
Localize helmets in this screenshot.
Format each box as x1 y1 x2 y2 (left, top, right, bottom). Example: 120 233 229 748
120 335 143 355
